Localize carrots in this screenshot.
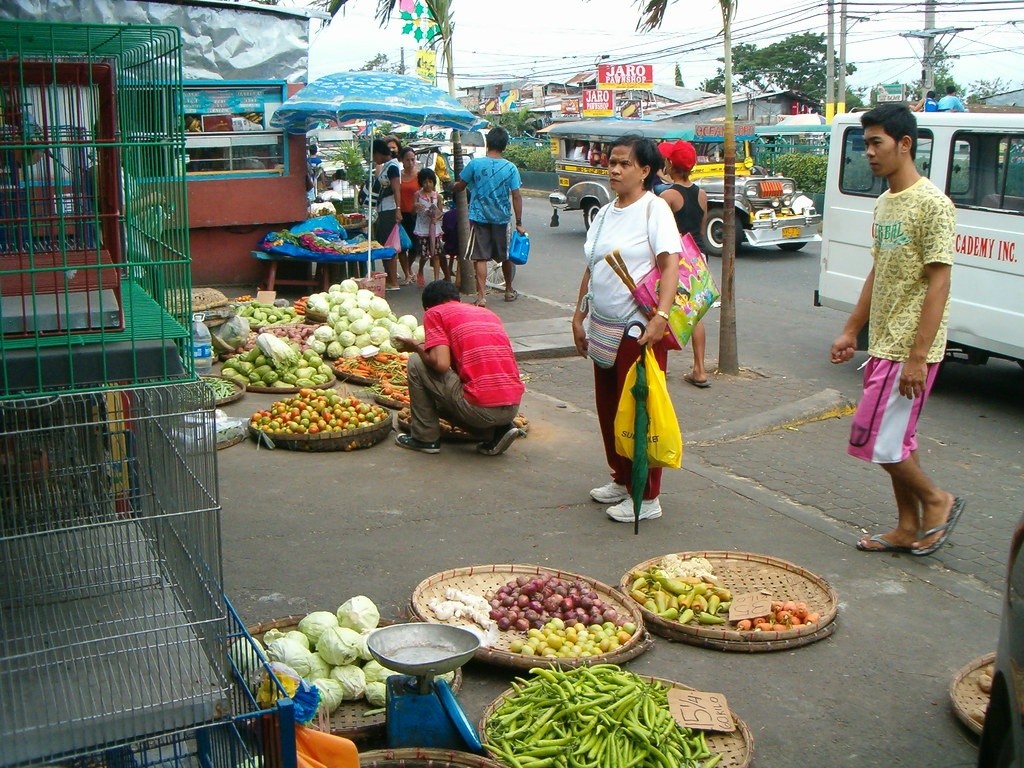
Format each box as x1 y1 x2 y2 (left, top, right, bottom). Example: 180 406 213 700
293 296 309 314
333 353 473 433
736 601 820 631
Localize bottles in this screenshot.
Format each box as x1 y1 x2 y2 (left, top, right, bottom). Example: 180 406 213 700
508 230 530 265
184 313 212 374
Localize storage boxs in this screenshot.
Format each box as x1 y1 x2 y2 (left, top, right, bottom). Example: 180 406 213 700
357 271 388 300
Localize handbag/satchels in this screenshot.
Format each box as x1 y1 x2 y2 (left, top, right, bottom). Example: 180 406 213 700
615 342 683 469
383 220 412 253
630 199 720 350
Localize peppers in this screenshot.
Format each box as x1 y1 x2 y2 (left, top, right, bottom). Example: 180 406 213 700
479 662 724 768
623 564 732 625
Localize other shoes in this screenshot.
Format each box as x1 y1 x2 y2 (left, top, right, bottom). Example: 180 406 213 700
416 271 424 288
400 267 416 286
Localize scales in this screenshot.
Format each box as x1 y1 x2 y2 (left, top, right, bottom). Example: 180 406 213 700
366 622 485 756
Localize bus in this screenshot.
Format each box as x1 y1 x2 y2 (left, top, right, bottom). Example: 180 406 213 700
536 120 823 256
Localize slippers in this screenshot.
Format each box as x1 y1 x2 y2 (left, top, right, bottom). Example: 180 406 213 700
385 283 400 291
857 528 912 552
684 370 711 387
913 494 965 558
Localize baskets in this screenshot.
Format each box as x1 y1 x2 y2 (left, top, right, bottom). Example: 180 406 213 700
164 286 529 453
228 550 840 768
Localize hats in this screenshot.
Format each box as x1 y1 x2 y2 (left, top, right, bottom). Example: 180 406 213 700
658 137 696 171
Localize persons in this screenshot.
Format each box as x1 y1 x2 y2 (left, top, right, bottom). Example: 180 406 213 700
413 168 472 287
572 134 682 522
84 119 165 288
307 145 326 189
575 141 610 166
331 169 350 197
369 137 420 289
830 104 966 557
909 86 969 112
451 128 525 306
654 158 674 195
658 140 711 387
395 280 524 455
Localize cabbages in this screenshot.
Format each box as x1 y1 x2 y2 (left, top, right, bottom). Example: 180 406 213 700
257 332 298 368
227 595 456 718
304 279 427 359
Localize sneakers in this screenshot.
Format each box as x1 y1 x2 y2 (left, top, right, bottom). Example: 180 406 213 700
607 492 662 523
394 431 441 453
589 481 632 503
476 421 519 455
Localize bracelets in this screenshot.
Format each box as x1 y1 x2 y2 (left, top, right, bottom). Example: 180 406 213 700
397 207 400 209
516 219 521 224
656 311 669 321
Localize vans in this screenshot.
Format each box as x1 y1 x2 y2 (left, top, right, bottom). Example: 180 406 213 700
423 128 488 170
813 103 1024 372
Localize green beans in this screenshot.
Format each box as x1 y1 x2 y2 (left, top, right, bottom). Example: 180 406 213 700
201 377 237 401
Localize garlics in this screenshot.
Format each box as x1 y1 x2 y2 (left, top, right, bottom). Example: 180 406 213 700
429 587 492 630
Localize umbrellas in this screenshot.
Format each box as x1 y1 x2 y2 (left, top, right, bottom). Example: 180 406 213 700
623 321 649 535
269 71 489 278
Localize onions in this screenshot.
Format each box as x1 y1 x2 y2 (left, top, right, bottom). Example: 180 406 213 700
485 573 630 632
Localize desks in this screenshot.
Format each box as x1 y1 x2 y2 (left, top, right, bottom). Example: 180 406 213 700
249 248 396 295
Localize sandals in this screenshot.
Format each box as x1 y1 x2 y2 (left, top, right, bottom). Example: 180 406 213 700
472 298 486 308
504 288 517 302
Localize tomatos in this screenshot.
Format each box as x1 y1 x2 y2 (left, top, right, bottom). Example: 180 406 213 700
508 618 634 660
250 388 390 435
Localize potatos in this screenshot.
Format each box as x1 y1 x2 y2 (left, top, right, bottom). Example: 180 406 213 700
223 322 321 358
